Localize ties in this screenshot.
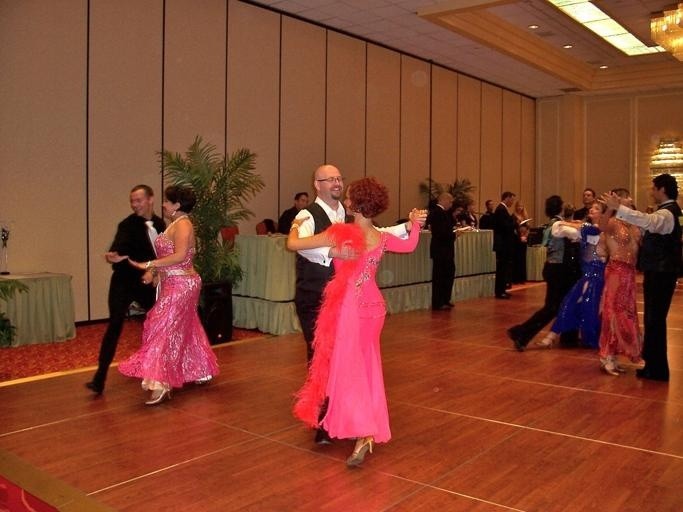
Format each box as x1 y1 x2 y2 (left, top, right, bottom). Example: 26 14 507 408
144 220 158 258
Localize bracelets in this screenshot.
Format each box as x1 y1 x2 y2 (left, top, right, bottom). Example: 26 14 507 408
152 269 156 274
290 224 300 230
145 261 154 268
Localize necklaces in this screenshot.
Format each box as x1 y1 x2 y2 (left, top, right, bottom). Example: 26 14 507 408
162 215 189 233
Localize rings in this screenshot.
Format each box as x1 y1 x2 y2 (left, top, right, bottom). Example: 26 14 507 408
143 281 145 283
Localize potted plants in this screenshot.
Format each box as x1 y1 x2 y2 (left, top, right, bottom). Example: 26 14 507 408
153 136 266 344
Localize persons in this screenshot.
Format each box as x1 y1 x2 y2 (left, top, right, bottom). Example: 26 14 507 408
86 184 167 394
287 176 428 466
293 163 429 445
452 191 531 298
117 186 220 405
428 192 462 310
278 192 309 234
507 174 683 383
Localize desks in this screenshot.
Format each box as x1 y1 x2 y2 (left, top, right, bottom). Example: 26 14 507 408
526 246 547 282
1 271 76 349
224 227 494 336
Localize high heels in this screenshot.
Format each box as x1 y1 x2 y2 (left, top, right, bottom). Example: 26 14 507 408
600 357 619 377
346 435 373 467
144 385 171 405
616 363 625 372
536 336 555 349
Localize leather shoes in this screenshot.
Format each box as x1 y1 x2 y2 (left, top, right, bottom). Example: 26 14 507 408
506 329 524 352
314 430 331 445
636 369 669 382
433 303 455 311
496 283 513 300
85 379 105 394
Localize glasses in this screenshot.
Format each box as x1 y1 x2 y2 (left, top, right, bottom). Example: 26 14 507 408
317 176 345 182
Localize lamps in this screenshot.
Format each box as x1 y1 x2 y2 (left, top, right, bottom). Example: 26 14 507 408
650 3 683 63
649 138 682 216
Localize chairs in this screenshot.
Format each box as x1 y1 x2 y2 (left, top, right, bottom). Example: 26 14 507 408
221 224 240 250
255 222 278 235
